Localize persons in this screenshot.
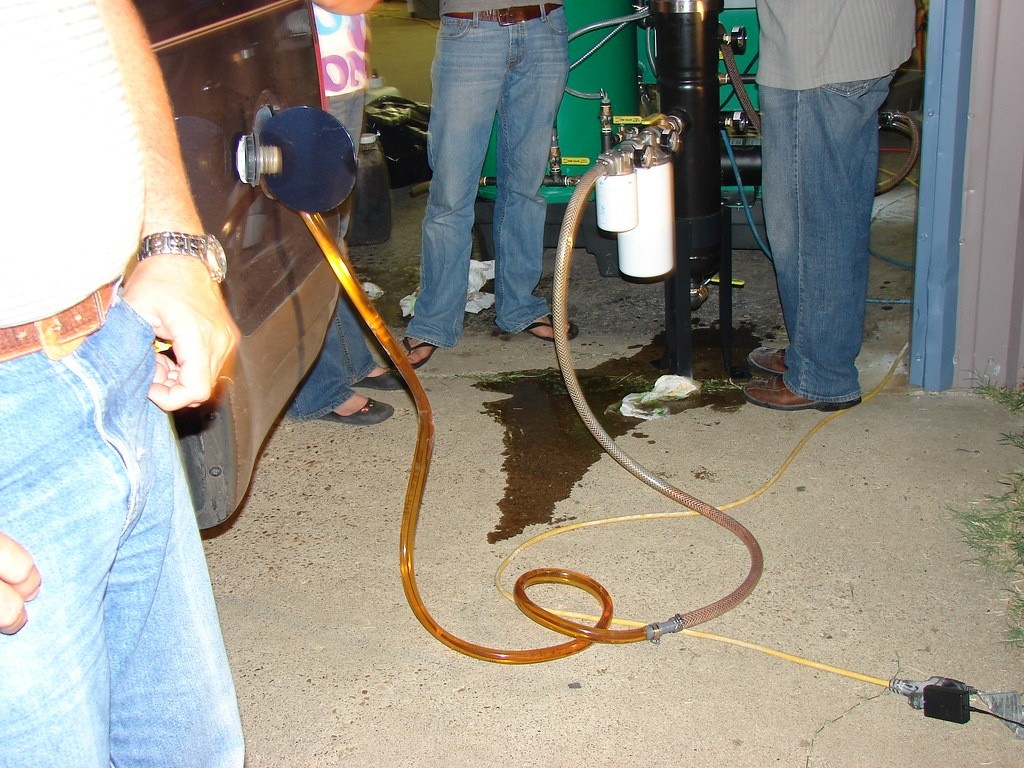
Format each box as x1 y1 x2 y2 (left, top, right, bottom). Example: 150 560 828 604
0 0 246 768
288 0 409 425
397 0 578 369
738 0 916 413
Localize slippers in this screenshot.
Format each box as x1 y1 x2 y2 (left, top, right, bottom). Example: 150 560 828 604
525 314 579 341
398 336 437 369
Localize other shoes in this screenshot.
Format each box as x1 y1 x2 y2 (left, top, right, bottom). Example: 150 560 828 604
318 394 394 426
351 368 408 390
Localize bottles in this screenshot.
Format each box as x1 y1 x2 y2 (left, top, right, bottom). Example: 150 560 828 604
344 133 393 248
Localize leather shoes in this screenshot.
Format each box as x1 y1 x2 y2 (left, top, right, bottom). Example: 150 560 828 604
742 374 861 412
748 346 788 376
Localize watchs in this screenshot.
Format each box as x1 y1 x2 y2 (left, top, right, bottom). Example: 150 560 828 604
138 231 228 284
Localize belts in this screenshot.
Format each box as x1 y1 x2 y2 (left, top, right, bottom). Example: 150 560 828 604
444 3 563 26
0 282 115 364
